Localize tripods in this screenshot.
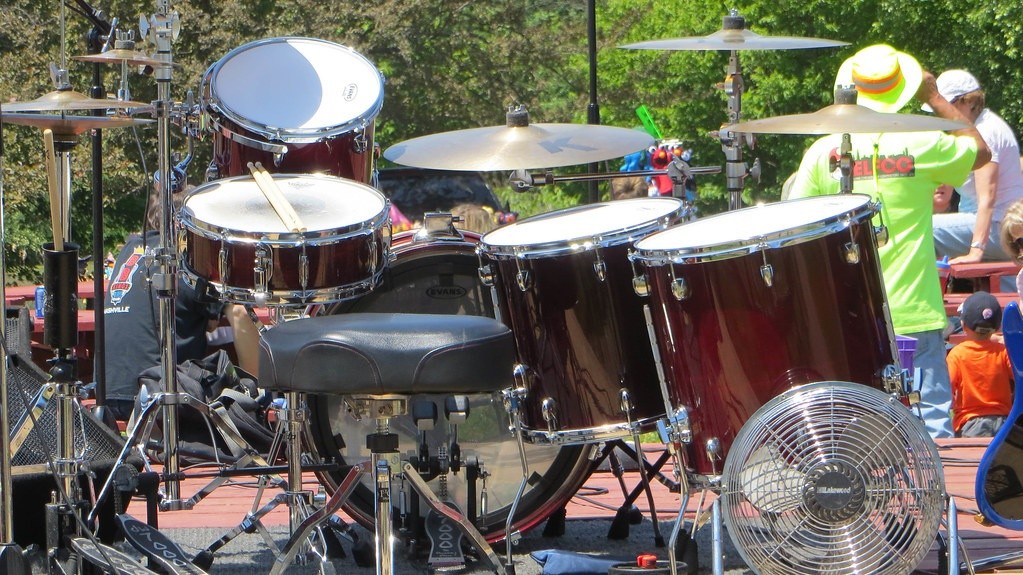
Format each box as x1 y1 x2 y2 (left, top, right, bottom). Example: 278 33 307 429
51 0 378 575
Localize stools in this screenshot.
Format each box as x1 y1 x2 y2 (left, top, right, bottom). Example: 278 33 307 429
258 313 515 575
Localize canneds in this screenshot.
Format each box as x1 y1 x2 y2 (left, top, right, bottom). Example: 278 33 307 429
35 286 45 319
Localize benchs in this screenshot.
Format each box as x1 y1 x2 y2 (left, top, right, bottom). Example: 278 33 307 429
939 259 1023 346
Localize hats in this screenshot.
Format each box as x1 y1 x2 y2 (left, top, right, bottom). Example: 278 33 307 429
921 70 979 113
834 44 923 112
956 291 1002 334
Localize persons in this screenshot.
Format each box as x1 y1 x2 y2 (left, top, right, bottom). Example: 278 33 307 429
789 45 993 438
94 183 279 464
946 290 1014 438
932 183 989 294
920 69 1023 259
1000 199 1023 314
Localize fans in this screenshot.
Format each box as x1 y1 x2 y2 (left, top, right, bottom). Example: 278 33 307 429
713 381 960 575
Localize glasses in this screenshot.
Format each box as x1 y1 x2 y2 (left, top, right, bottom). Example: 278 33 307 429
1008 237 1023 253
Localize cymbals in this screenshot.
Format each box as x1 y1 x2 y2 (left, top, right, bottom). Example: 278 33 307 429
2 87 153 113
381 121 658 173
615 30 858 53
69 49 179 66
723 103 976 136
1 114 157 137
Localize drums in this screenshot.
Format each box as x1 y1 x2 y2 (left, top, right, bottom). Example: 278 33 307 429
200 35 387 185
177 170 392 307
630 192 907 488
300 227 595 551
474 194 689 443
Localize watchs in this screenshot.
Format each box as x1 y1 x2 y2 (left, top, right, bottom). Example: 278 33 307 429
970 242 987 250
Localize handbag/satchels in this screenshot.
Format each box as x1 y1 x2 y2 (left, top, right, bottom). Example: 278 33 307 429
125 349 276 467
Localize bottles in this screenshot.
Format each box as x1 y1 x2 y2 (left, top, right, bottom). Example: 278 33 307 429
637 555 658 575
936 256 951 299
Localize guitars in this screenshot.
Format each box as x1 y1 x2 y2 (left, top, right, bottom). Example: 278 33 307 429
974 299 1023 532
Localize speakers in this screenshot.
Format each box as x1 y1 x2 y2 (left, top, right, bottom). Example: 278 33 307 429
0 349 145 558
4 304 32 361
